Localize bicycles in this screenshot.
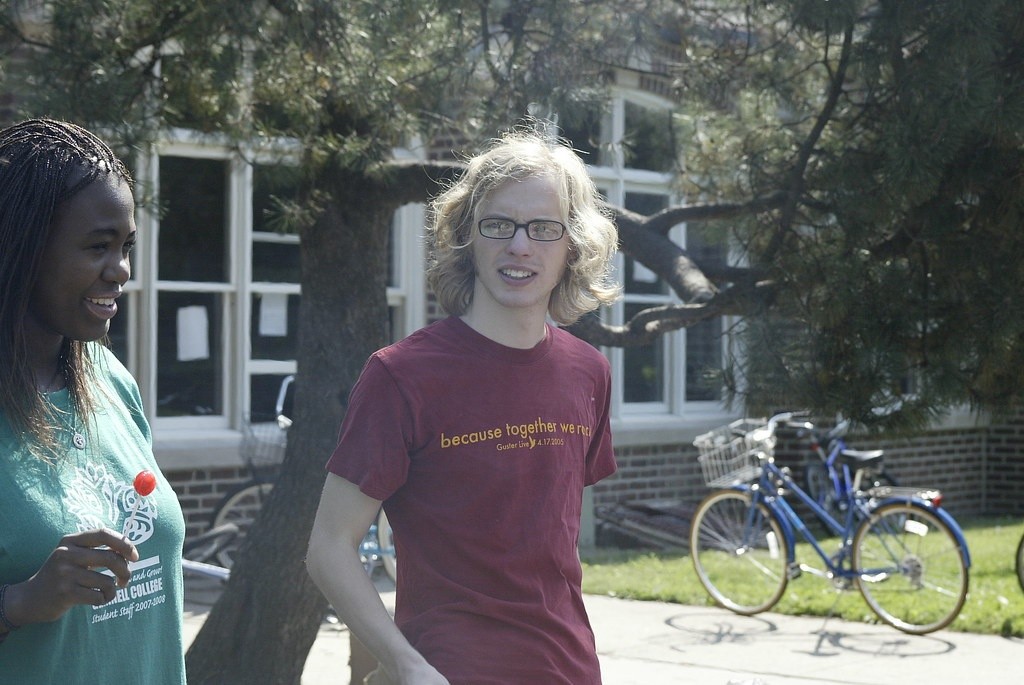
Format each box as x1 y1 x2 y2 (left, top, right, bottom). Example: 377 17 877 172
689 408 972 636
180 375 397 629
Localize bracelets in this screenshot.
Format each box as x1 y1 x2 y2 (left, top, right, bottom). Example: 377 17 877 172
0 584 19 630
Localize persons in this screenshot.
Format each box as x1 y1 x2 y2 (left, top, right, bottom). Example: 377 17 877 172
305 127 619 685
0 117 194 685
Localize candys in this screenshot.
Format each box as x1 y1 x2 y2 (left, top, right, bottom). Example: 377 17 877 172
124 470 156 537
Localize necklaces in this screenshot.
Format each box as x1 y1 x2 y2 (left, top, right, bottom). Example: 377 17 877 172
32 386 86 450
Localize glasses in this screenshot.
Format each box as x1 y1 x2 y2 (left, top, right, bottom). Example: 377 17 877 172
471 216 568 243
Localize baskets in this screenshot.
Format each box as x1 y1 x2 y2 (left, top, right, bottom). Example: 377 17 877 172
240 414 291 468
694 417 775 489
